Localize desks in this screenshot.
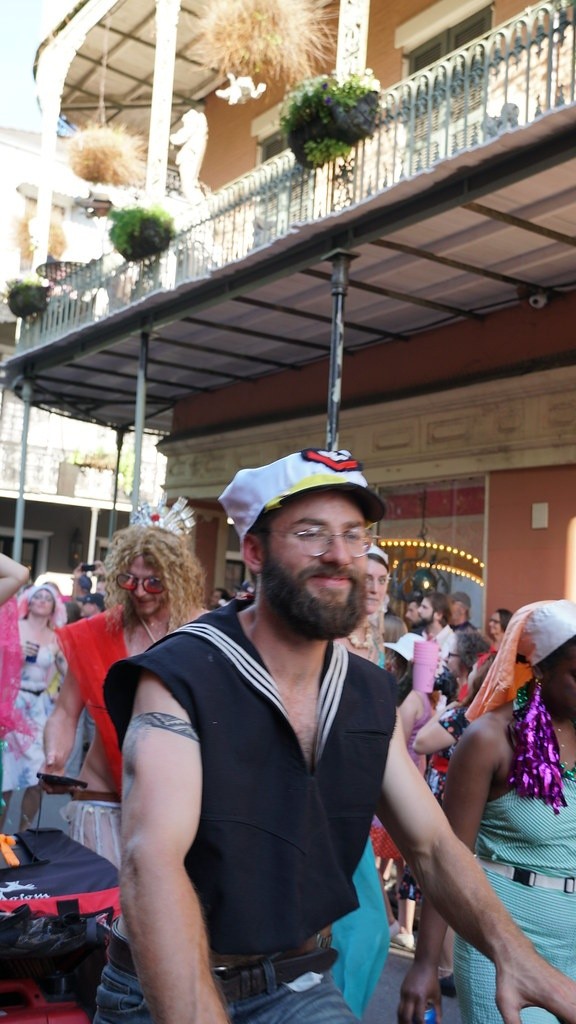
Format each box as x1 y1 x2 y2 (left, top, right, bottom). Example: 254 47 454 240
36 263 85 282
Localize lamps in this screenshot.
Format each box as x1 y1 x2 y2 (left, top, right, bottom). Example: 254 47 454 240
392 486 450 610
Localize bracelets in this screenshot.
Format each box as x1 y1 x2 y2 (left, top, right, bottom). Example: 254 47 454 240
316 933 332 948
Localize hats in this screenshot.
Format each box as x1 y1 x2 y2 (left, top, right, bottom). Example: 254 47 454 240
368 544 389 564
383 633 426 661
71 574 91 590
77 594 104 611
446 592 471 608
217 447 385 542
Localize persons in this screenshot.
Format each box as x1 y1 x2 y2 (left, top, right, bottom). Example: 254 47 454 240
300 542 390 1024
395 600 576 1024
369 591 512 948
170 109 208 202
0 549 105 836
208 580 255 609
37 508 211 866
103 451 576 1024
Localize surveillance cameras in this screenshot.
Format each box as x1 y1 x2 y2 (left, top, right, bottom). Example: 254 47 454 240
529 294 548 307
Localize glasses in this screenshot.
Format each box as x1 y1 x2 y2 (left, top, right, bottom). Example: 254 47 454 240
116 572 166 594
258 527 374 557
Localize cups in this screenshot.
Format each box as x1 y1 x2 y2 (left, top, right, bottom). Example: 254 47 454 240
413 641 439 693
27 642 39 663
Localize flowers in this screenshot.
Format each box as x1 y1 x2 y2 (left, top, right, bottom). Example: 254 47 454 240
2 277 42 297
276 68 383 131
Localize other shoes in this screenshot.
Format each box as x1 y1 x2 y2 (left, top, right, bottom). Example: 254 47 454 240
437 972 457 998
388 920 401 939
393 934 415 946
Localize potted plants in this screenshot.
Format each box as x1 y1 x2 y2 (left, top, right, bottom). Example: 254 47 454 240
109 202 174 262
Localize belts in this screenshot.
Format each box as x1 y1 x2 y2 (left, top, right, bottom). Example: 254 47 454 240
108 923 339 1002
476 857 576 894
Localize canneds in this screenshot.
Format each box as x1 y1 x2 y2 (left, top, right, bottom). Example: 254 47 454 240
413 1003 438 1024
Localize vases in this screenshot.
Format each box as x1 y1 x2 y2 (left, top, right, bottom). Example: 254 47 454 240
286 91 378 170
8 286 49 316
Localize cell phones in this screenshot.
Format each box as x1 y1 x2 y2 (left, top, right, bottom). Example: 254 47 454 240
37 773 88 788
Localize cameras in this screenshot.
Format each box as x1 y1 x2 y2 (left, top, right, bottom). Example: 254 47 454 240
433 671 459 695
81 564 95 571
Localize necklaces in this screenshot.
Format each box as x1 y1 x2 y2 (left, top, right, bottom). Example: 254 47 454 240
510 681 576 814
142 618 157 643
348 626 372 648
